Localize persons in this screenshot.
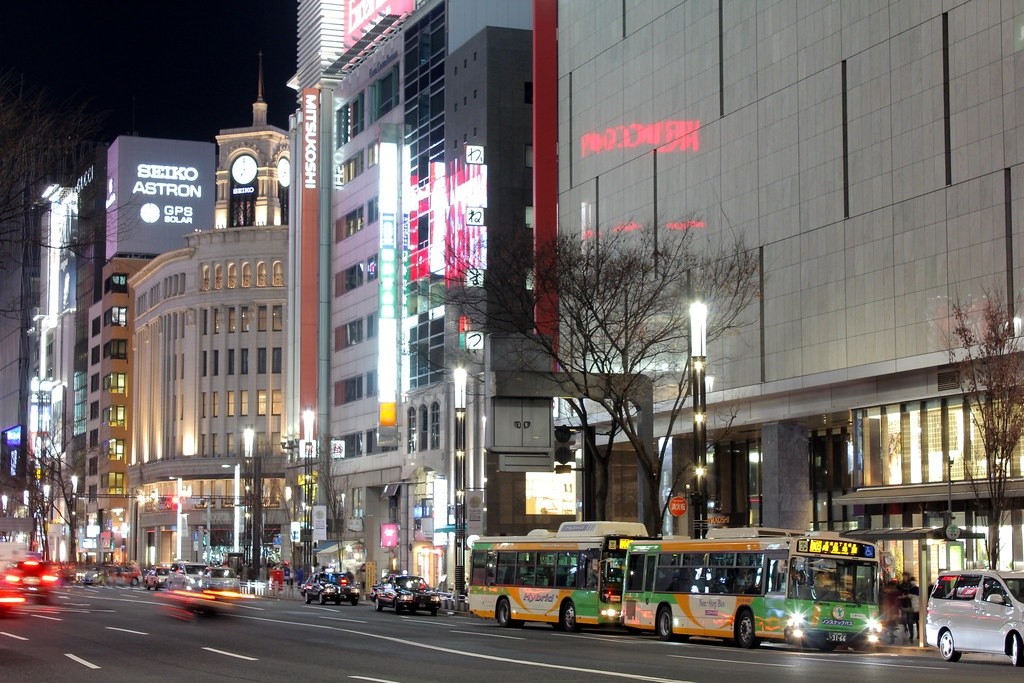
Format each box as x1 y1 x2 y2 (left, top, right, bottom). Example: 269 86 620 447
238 562 366 592
882 572 933 646
59 561 136 586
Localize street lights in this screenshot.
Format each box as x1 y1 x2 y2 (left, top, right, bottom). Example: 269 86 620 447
221 463 243 553
168 476 182 560
690 297 710 538
70 475 78 563
304 410 317 575
451 367 466 614
240 426 256 572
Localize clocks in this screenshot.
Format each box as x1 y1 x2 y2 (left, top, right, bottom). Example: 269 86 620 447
278 155 291 187
229 153 259 186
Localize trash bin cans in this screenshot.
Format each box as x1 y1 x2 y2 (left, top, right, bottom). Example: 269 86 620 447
437 591 447 610
458 595 465 611
445 593 454 610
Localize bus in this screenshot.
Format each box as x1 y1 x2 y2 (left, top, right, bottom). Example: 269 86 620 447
620 527 892 654
463 520 654 632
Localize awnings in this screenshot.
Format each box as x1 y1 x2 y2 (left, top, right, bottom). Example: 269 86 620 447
434 524 468 534
312 539 358 564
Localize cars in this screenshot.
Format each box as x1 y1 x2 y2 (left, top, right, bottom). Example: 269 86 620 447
195 566 242 595
0 552 144 614
143 565 172 591
370 575 442 616
299 571 362 606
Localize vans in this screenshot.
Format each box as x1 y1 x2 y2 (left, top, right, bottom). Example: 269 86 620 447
925 570 1024 668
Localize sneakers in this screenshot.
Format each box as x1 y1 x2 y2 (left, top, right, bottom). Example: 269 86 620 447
909 639 914 644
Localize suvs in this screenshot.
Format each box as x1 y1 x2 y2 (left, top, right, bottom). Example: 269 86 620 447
166 560 209 589
